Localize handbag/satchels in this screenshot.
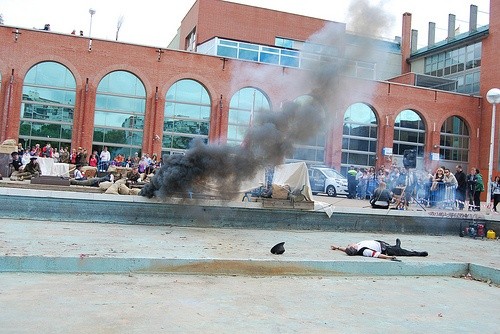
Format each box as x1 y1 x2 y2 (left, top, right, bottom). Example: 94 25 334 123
369 198 390 209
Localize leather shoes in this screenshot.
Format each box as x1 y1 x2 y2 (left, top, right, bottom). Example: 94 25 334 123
414 251 428 257
395 238 401 248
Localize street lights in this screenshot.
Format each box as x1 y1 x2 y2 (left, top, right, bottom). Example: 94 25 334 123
485 88 500 207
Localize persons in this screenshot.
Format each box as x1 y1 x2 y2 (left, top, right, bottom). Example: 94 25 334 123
74 164 89 180
347 164 484 211
18 143 163 175
23 157 42 179
9 152 25 180
491 176 500 212
331 239 428 260
370 181 392 209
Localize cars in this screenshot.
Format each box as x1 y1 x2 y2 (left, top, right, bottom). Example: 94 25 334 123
308 167 349 197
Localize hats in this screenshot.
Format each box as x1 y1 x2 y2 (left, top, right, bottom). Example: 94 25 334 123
270 242 286 255
